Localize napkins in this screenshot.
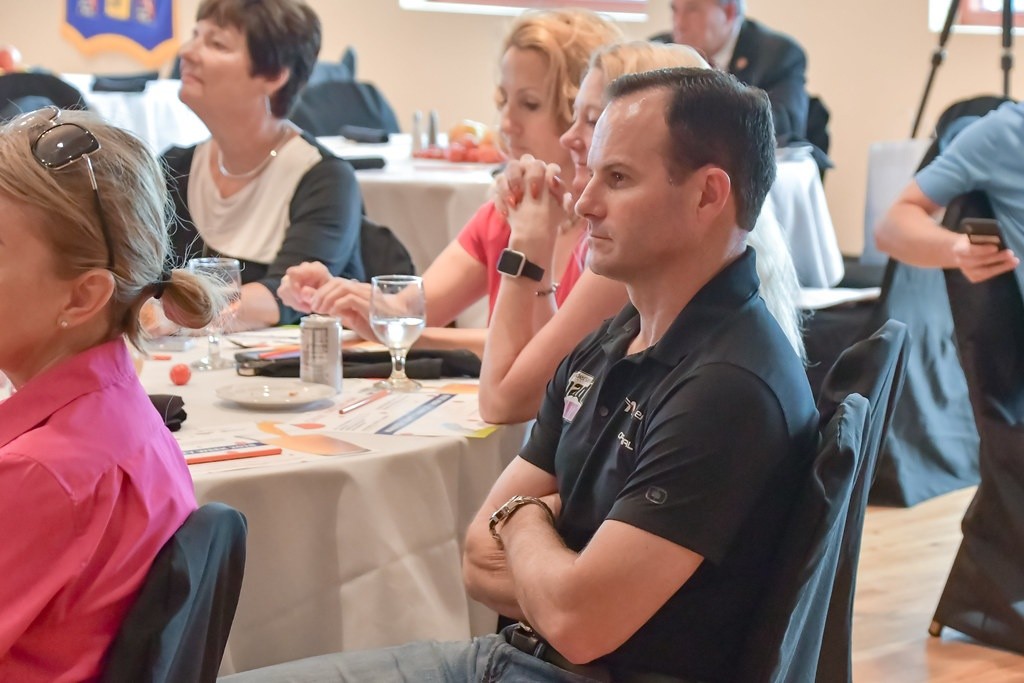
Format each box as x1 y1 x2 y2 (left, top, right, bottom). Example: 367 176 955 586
256 350 481 382
150 393 190 428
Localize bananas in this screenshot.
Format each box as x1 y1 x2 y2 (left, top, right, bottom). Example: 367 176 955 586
447 119 493 144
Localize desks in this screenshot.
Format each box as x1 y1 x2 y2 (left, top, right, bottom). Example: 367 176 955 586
68 75 215 163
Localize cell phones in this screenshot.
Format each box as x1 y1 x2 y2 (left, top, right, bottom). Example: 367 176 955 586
962 218 1010 253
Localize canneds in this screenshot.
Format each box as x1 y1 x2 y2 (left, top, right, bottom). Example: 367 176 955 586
299 314 343 394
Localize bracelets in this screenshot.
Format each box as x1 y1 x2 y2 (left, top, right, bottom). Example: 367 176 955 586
535 283 558 296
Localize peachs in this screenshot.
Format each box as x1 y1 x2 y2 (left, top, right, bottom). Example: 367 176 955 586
448 134 507 164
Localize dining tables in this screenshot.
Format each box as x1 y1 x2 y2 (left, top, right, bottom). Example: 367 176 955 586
317 130 849 372
119 314 539 678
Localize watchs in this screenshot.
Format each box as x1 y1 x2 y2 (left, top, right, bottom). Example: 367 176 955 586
489 495 554 549
496 248 545 282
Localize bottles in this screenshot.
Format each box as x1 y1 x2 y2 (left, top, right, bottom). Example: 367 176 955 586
412 110 423 155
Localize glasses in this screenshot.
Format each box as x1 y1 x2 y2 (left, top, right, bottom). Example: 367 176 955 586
10 105 115 271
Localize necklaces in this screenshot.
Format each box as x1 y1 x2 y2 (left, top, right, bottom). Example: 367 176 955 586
219 123 292 177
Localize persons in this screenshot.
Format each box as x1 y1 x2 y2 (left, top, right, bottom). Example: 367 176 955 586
0 107 200 682
874 98 1024 297
138 1 367 340
648 0 808 146
476 41 810 423
217 68 821 682
277 9 630 362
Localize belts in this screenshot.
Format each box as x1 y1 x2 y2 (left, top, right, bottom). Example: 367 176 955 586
510 628 613 683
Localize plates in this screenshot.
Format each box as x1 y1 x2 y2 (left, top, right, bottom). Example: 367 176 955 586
412 159 507 171
216 377 336 412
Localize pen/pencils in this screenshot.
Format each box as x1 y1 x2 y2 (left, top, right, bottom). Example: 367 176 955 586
260 345 302 357
151 354 171 360
339 390 390 414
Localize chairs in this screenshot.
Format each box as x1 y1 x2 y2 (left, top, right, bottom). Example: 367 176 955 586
751 318 911 681
806 95 1024 654
91 504 249 683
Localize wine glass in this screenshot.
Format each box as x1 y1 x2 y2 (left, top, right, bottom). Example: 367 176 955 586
188 258 237 370
370 275 423 393
